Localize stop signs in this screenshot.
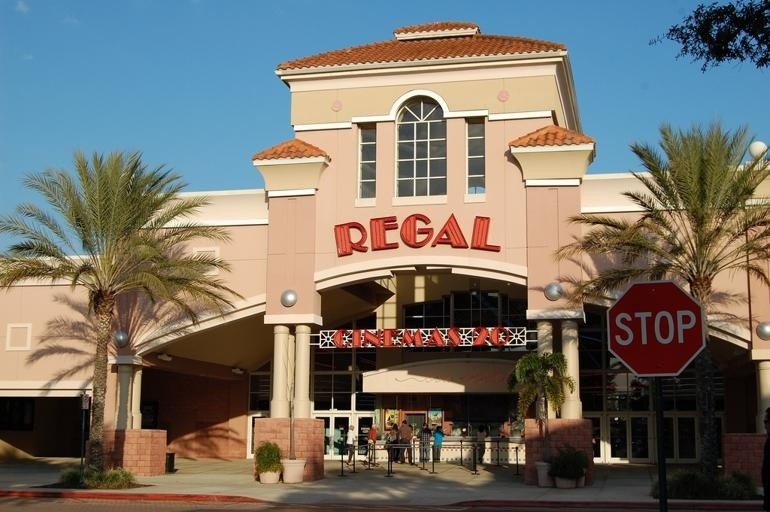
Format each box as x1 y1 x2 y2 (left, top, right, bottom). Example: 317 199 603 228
606 281 708 379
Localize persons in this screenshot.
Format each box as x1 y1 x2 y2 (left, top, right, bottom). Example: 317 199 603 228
387 419 444 465
364 424 378 465
476 428 487 463
346 425 358 467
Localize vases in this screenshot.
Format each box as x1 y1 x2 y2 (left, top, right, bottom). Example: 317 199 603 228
280 458 306 483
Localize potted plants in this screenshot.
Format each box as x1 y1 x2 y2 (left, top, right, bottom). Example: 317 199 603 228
255 439 283 484
547 443 589 488
506 351 576 488
